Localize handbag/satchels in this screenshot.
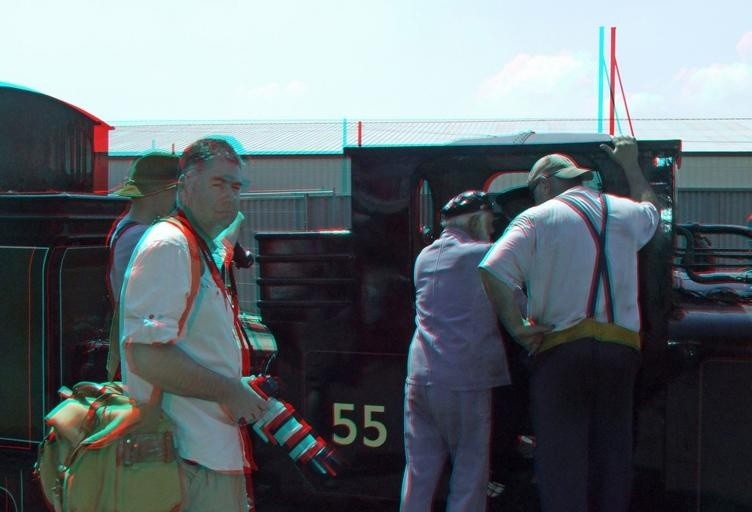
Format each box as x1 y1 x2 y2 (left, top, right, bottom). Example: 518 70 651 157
34 378 184 512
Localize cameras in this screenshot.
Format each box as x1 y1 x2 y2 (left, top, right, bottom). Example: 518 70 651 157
230 240 253 268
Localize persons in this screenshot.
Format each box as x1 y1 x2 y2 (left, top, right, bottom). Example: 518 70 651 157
398 191 528 512
105 150 237 382
118 137 276 512
475 133 662 512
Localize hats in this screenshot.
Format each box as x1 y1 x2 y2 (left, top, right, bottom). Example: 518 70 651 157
526 152 592 192
115 152 181 199
441 190 495 217
